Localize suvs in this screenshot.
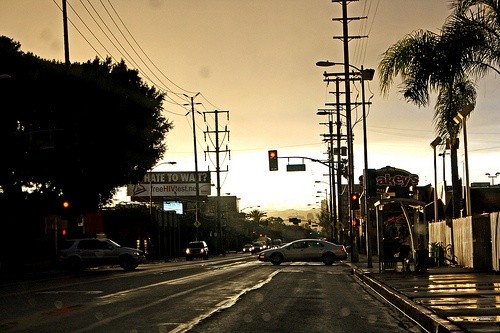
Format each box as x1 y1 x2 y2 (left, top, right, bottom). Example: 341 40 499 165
58 237 149 271
185 241 209 261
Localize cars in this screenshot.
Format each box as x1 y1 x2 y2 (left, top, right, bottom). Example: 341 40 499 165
258 239 349 266
242 237 282 255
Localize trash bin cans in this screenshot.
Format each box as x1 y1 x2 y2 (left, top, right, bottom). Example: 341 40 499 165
413 249 429 276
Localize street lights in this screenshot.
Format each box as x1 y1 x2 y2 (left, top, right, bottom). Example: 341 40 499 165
149 161 177 215
430 136 443 223
315 60 374 269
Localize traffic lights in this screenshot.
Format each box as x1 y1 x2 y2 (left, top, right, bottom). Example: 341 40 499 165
268 150 278 171
61 228 67 237
353 219 360 226
350 194 360 210
60 200 71 220
